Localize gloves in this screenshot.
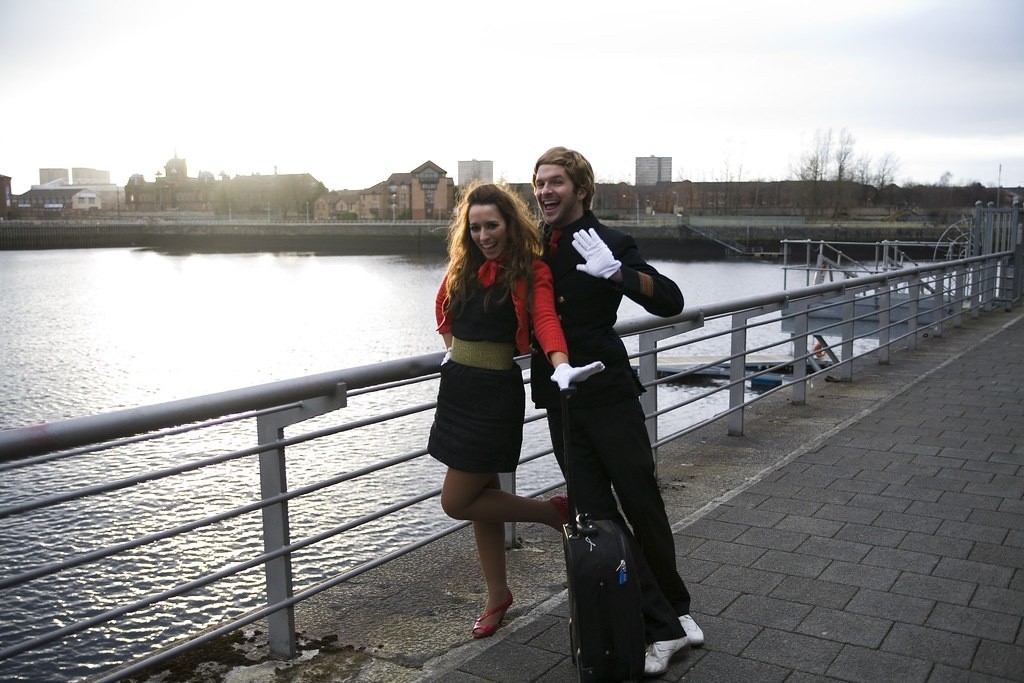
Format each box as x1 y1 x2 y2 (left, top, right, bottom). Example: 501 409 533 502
571 227 622 280
439 346 452 366
550 361 605 392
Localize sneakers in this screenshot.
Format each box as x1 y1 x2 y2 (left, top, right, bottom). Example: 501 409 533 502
676 614 704 646
643 635 691 675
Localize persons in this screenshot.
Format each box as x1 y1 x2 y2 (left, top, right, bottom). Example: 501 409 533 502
426 177 607 638
525 146 705 676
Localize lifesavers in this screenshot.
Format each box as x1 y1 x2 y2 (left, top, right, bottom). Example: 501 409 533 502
814 343 826 357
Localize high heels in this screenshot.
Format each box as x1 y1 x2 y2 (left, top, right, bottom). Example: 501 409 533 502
469 586 513 638
550 493 570 521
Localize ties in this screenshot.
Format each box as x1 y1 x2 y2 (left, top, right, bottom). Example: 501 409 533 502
547 229 562 256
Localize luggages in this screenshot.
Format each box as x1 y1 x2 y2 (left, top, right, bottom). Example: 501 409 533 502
561 387 645 683
